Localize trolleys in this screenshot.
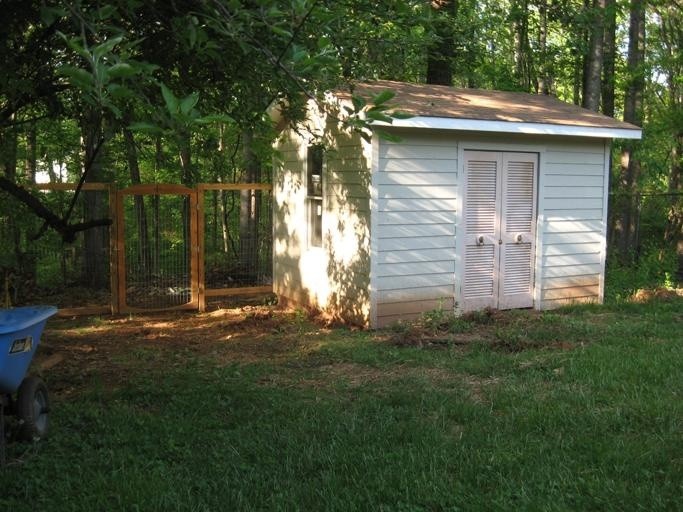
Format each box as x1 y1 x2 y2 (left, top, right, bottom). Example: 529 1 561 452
0 299 62 445
